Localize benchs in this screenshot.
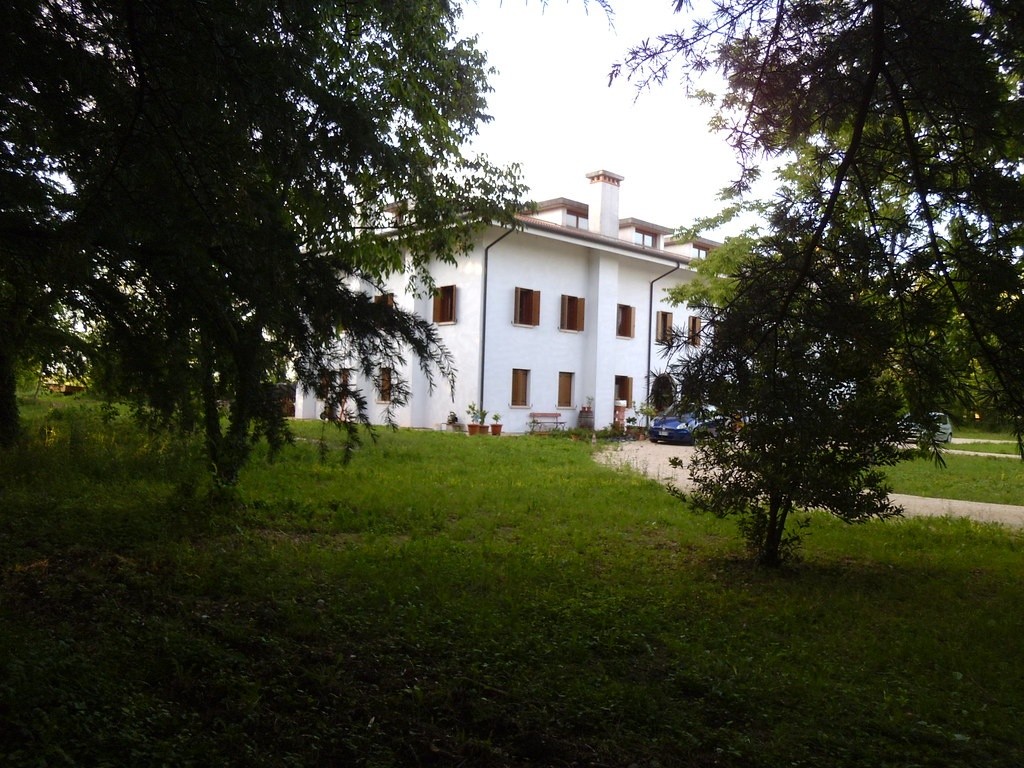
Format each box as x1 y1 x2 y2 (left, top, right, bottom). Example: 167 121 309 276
530 413 567 431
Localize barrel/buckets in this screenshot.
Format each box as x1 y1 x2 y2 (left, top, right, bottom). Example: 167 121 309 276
577 411 594 430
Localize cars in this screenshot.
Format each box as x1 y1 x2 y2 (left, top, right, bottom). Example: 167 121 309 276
897 411 952 444
647 402 732 445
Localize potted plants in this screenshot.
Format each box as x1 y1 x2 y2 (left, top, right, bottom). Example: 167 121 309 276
491 413 503 435
626 401 656 441
466 401 489 435
